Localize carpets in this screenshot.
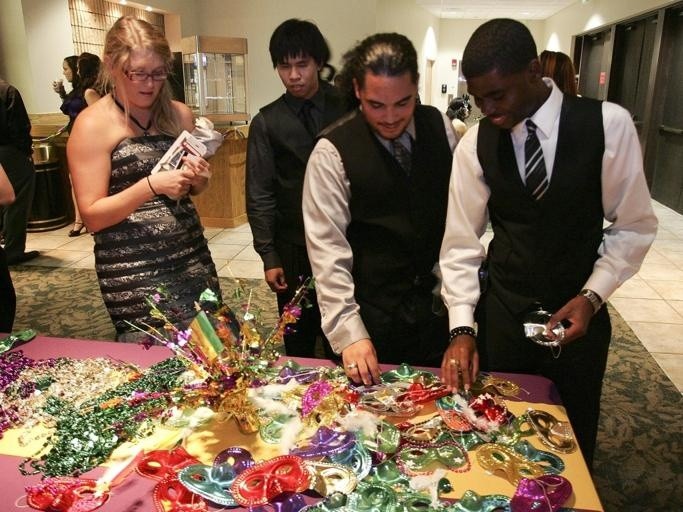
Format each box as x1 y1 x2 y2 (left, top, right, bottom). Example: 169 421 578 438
1 263 681 493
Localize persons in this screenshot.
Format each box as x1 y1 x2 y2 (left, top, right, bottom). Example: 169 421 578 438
52 56 80 133
539 48 584 97
0 166 17 339
0 81 40 265
248 18 351 358
432 17 659 477
60 52 107 238
445 97 472 140
301 33 456 385
64 15 225 343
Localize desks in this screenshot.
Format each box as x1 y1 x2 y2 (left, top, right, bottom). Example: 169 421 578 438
1 329 604 512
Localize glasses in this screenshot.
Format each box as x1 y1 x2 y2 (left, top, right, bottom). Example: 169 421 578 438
120 66 169 82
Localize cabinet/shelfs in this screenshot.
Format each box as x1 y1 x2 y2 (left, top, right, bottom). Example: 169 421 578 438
180 34 252 127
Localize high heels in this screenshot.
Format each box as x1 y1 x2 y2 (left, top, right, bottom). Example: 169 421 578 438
68 222 88 237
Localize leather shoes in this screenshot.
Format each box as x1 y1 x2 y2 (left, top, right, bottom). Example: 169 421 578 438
5 250 40 266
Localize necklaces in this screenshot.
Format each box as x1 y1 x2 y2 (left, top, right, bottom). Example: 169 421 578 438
107 93 158 138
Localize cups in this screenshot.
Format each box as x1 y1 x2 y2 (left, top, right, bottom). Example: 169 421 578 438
55 79 62 92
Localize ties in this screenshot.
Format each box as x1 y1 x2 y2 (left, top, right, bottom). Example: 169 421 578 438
524 119 550 203
391 138 411 175
300 99 317 139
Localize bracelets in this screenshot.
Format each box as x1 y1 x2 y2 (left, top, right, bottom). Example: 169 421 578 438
578 288 600 316
447 326 477 343
144 176 158 196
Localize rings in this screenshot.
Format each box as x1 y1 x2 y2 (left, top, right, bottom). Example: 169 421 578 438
450 358 461 365
346 360 357 369
